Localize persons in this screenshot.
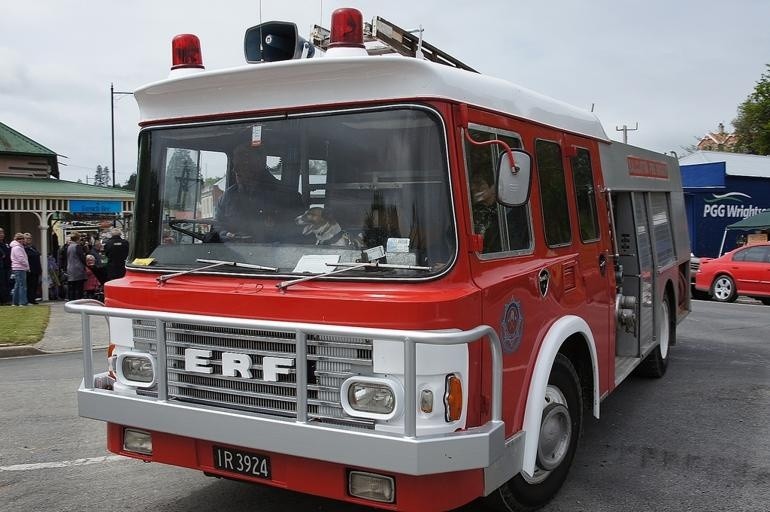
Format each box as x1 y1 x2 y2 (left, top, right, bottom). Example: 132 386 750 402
0 225 130 307
447 172 531 252
210 140 303 243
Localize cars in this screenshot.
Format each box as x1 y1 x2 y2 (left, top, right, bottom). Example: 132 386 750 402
696 240 770 304
688 251 714 298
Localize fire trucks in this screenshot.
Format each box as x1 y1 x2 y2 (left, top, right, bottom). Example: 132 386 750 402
69 11 694 512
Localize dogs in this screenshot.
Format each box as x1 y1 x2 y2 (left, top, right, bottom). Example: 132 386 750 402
293 206 354 247
